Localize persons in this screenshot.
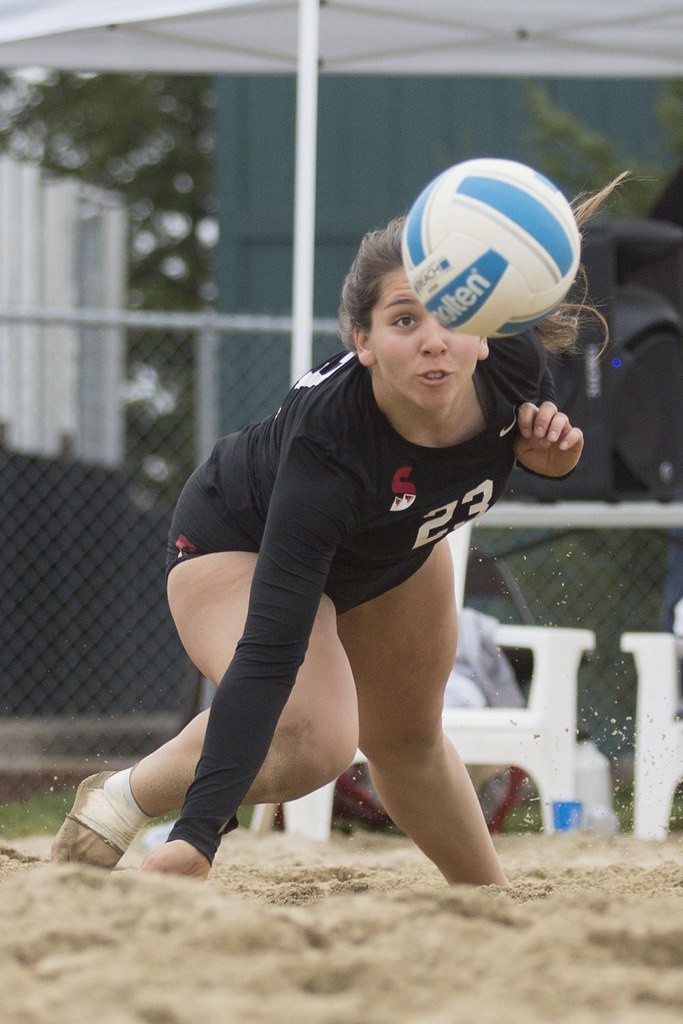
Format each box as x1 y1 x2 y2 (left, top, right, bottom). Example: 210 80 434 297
51 170 637 890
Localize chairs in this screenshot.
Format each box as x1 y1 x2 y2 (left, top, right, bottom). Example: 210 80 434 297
251 520 595 841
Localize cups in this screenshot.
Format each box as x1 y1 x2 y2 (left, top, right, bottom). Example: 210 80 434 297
555 802 581 830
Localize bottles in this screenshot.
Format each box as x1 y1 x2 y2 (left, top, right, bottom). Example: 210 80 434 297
573 731 617 833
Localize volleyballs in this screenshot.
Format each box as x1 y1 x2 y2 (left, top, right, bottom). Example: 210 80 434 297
400 152 583 340
